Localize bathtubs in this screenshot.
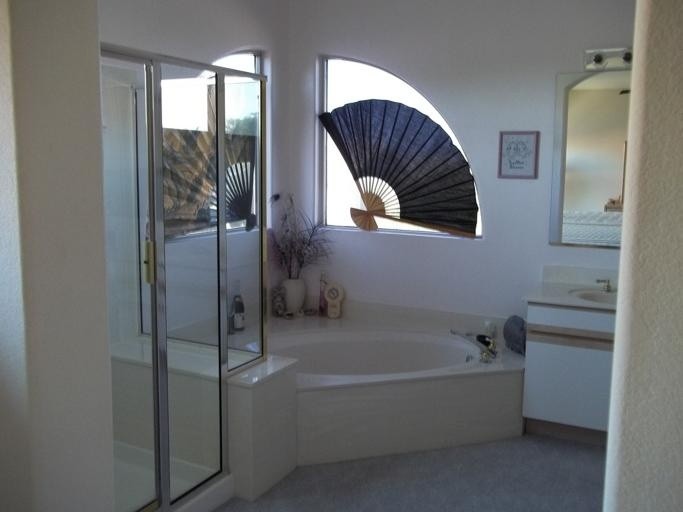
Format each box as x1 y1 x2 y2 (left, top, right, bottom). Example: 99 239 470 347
204 314 523 448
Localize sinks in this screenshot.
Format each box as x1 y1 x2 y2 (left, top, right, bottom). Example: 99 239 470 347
577 290 615 306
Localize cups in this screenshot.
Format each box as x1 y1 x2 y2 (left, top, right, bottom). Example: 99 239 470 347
282 309 315 321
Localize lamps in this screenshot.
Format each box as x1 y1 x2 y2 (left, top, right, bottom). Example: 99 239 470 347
584 46 633 71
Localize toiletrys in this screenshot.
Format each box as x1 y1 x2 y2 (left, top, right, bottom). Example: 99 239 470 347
315 269 330 317
231 290 249 332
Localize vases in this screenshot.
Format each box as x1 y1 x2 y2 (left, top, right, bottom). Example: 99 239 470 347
280 278 307 313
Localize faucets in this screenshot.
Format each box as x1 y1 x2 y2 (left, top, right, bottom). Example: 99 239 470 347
595 278 611 291
447 327 494 355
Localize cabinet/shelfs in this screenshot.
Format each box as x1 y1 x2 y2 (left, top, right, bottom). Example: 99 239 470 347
521 302 615 446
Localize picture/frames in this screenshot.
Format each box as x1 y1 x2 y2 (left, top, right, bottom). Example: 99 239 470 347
497 131 540 180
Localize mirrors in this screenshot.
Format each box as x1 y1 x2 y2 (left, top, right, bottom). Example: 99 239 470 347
547 69 633 250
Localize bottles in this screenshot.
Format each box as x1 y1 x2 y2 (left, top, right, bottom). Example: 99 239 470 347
319 271 328 313
232 280 244 331
